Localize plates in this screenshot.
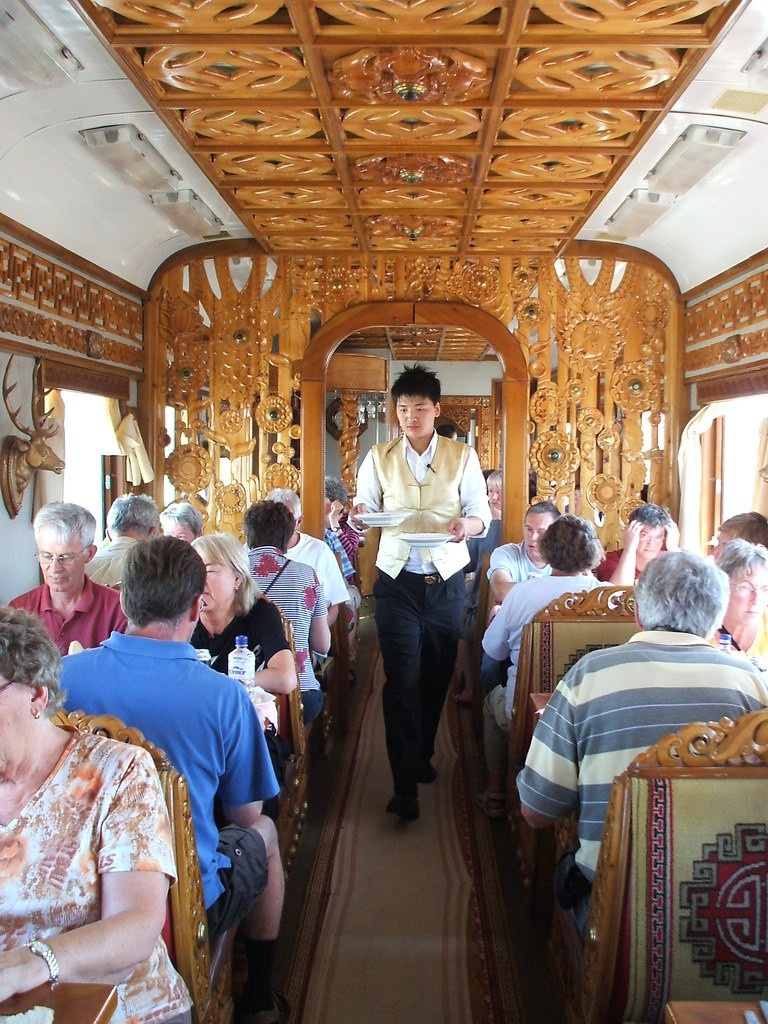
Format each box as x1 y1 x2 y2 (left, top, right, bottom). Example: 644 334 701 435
397 534 455 549
354 513 412 527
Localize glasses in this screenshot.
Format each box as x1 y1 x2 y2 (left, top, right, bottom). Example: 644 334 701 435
730 581 768 600
33 545 91 567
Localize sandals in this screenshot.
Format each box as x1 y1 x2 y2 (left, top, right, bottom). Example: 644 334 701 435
475 789 508 817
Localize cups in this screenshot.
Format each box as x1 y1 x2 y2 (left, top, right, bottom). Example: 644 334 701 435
195 649 211 668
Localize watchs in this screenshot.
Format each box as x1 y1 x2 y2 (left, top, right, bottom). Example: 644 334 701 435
23 940 59 988
331 527 341 532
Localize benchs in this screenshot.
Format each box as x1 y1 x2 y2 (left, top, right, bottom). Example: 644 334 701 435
476 554 768 1024
43 535 366 1024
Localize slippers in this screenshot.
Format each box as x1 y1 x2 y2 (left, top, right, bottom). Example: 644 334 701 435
456 670 465 682
452 694 473 708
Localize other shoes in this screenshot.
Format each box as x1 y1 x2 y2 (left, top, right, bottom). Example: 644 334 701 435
418 734 439 783
385 788 420 821
235 992 291 1024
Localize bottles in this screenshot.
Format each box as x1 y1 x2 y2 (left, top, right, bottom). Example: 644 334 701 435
228 636 255 703
718 634 733 659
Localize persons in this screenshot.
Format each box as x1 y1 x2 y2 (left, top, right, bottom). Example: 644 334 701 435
344 361 493 818
0 477 360 1023
439 424 768 906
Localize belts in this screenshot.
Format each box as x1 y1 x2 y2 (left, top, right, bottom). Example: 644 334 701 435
401 569 444 584
